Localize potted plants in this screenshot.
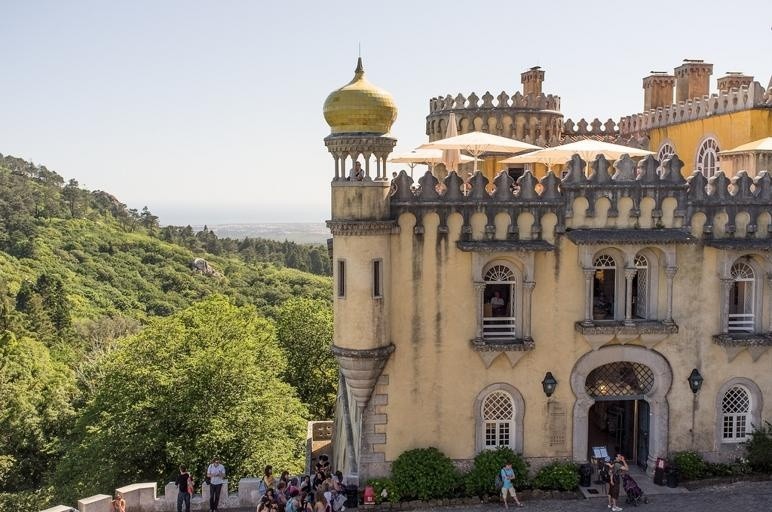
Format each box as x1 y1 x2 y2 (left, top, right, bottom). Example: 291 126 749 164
594 301 607 320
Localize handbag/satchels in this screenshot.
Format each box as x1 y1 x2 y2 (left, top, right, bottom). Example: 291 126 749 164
204 473 211 485
494 474 503 491
187 477 194 495
258 480 347 511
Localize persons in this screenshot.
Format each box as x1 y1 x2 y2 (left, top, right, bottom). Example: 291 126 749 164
111 492 126 512
601 453 629 512
255 454 347 512
175 465 193 512
490 291 504 305
500 462 524 510
207 458 226 512
348 161 364 180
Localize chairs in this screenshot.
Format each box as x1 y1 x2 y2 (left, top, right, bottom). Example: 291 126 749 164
484 302 493 318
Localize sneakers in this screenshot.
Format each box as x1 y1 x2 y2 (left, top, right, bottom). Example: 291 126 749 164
607 504 623 512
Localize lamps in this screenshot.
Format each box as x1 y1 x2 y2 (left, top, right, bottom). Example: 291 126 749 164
687 365 705 394
542 373 557 397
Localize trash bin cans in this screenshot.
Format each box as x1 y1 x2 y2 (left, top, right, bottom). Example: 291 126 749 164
578 465 594 486
346 485 357 508
664 465 681 488
